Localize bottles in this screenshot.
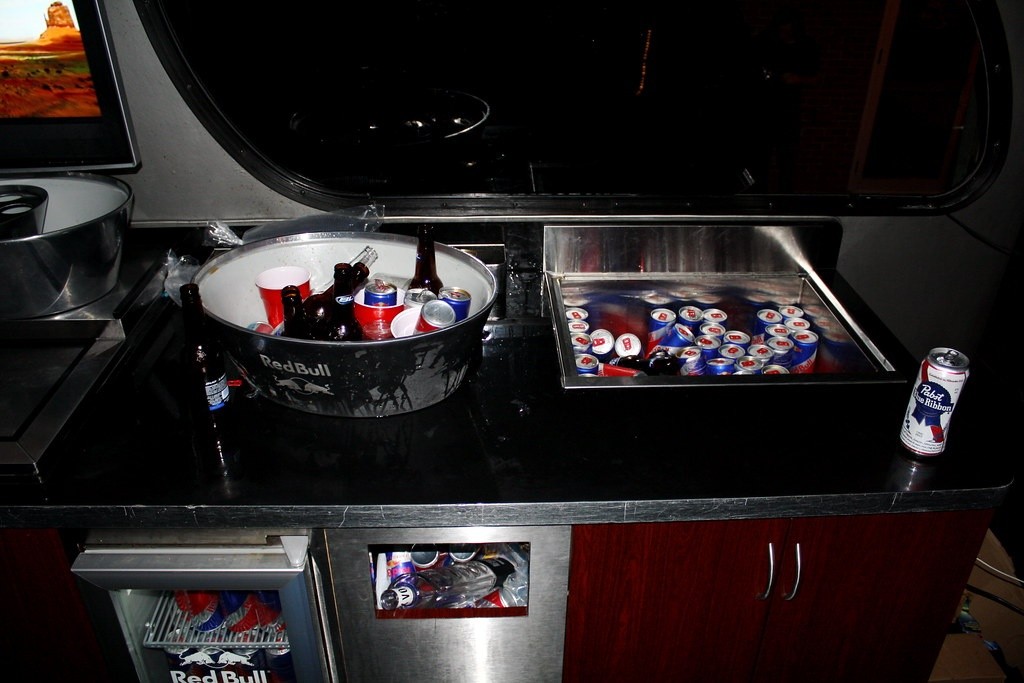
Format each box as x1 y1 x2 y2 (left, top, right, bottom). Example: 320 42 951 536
407 223 443 293
178 282 231 418
270 244 378 343
381 557 515 609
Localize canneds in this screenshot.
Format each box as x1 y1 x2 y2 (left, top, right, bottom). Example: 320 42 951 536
900 347 970 456
893 455 932 493
462 588 519 608
246 320 274 334
162 589 298 683
363 279 471 336
566 305 818 375
384 544 479 590
563 294 797 340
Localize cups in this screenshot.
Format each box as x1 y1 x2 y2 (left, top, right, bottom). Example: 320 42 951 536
352 288 405 342
254 266 311 328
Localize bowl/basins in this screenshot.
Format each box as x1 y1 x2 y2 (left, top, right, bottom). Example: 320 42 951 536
0 172 135 318
286 84 491 171
191 232 499 417
